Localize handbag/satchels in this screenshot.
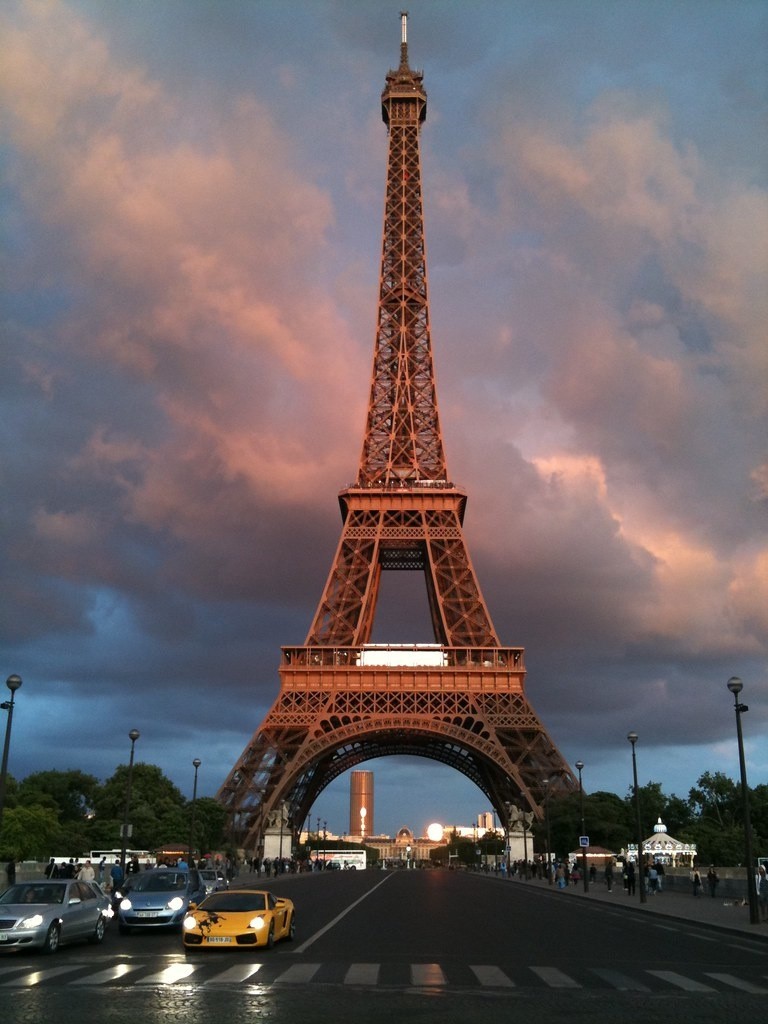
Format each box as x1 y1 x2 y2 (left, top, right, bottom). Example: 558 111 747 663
623 873 628 880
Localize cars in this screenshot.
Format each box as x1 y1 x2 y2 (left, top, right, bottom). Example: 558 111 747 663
113 873 145 912
198 869 229 894
0 880 114 955
119 868 206 934
91 881 113 906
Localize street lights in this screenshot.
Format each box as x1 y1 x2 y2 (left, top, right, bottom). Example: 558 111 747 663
483 813 488 875
120 728 140 871
0 673 23 831
188 758 202 868
543 779 553 885
627 731 648 904
727 675 762 926
521 791 529 880
575 759 590 892
231 776 327 870
505 801 511 878
493 808 498 876
473 823 476 872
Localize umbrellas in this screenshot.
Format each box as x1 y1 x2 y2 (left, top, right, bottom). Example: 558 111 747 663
214 853 222 861
204 853 212 858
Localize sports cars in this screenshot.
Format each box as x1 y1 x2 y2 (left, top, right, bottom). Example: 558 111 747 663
183 890 296 949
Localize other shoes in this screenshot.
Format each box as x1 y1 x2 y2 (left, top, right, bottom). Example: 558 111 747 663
608 890 612 893
623 888 628 890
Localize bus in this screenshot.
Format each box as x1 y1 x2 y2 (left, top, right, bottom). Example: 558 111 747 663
89 849 152 866
310 849 367 871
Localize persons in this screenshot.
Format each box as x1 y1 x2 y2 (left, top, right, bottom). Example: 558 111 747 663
689 865 702 896
605 861 614 892
707 867 719 898
24 888 37 902
5 858 15 885
755 865 768 922
644 860 665 896
53 888 64 903
110 860 123 898
43 855 324 886
621 863 636 896
497 858 583 888
589 863 596 883
169 874 186 889
